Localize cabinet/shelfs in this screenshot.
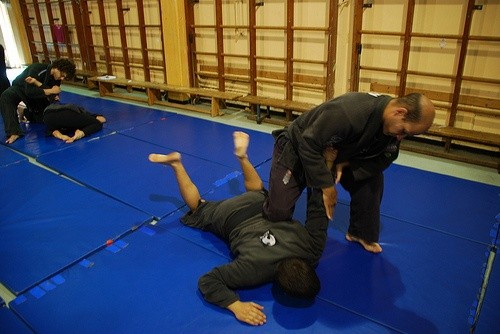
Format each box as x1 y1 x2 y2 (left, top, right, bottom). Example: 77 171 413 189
184 0 338 108
19 0 86 71
79 0 166 85
349 0 500 127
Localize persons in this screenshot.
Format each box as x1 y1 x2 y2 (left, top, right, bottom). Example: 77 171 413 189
0 44 106 144
261 93 436 252
148 131 339 327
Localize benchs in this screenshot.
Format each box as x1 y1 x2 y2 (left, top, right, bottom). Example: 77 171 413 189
88 75 242 117
239 94 500 174
62 70 107 90
